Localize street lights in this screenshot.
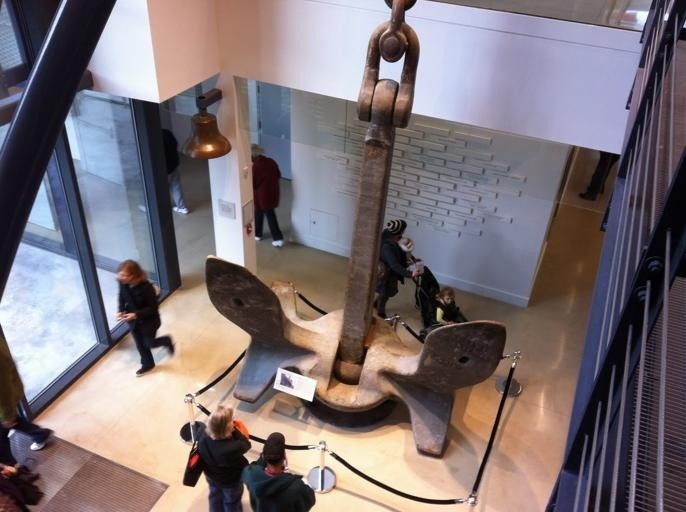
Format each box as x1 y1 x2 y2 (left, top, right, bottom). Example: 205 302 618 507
182 432 204 487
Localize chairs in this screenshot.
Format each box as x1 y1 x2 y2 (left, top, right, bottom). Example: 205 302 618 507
165 336 174 354
135 363 155 376
255 236 262 241
30 429 55 452
172 206 189 214
272 240 282 247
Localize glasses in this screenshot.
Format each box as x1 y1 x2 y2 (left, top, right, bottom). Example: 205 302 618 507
180 89 233 160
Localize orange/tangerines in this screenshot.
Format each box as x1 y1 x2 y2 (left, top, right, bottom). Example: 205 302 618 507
410 258 472 334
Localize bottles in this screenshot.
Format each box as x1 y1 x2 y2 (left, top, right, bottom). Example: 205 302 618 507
387 218 407 235
262 432 285 464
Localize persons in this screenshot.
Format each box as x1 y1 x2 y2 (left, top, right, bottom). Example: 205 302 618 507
0 337 54 478
373 218 415 318
138 122 189 216
251 143 284 249
437 286 462 325
196 406 249 511
241 432 315 512
0 461 43 512
115 259 173 376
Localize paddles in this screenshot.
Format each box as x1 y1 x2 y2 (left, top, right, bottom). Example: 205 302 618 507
7 430 170 512
